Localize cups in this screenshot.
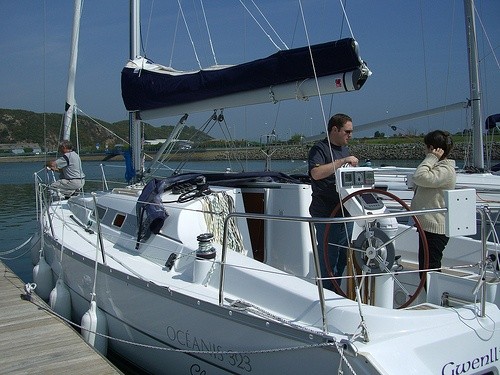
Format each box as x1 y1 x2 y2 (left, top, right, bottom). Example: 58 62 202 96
404 174 414 189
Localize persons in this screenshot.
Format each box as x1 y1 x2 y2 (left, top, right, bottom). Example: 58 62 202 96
45 139 86 201
409 130 456 292
308 114 359 294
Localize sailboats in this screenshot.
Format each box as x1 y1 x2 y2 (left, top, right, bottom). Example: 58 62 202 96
30 0 500 375
